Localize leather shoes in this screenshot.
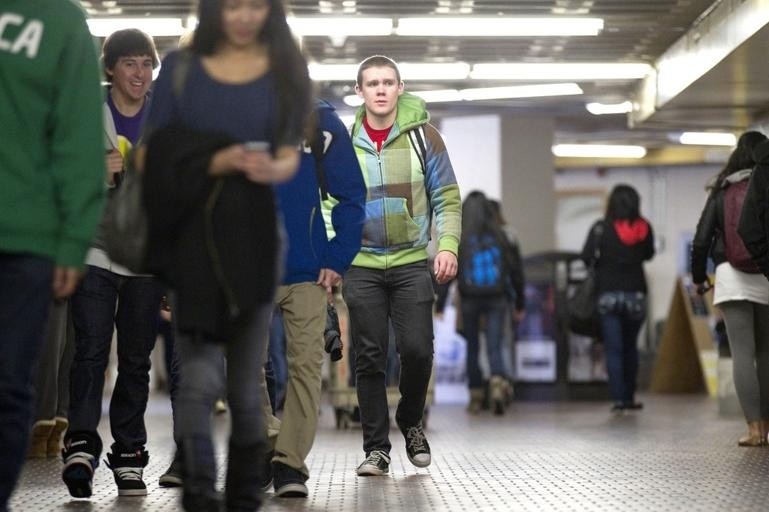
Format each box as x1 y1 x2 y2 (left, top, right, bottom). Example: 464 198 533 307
738 435 763 447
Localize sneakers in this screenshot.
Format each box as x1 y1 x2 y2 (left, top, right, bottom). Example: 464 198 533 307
102 446 148 497
158 453 183 486
402 418 431 467
273 467 308 496
258 449 273 491
61 437 95 499
355 449 391 476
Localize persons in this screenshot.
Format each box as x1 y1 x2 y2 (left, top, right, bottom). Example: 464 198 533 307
581 185 654 416
737 138 769 282
478 198 520 410
435 191 527 415
1 1 401 512
690 131 769 447
318 55 464 476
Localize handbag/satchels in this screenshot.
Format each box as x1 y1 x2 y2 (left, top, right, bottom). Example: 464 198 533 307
110 48 193 274
569 277 598 336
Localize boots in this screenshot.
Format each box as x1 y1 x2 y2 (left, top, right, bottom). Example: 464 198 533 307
47 417 68 456
27 420 57 459
487 374 506 416
467 387 485 414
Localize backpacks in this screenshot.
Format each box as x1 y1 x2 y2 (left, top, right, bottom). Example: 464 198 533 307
723 177 761 273
457 232 508 298
